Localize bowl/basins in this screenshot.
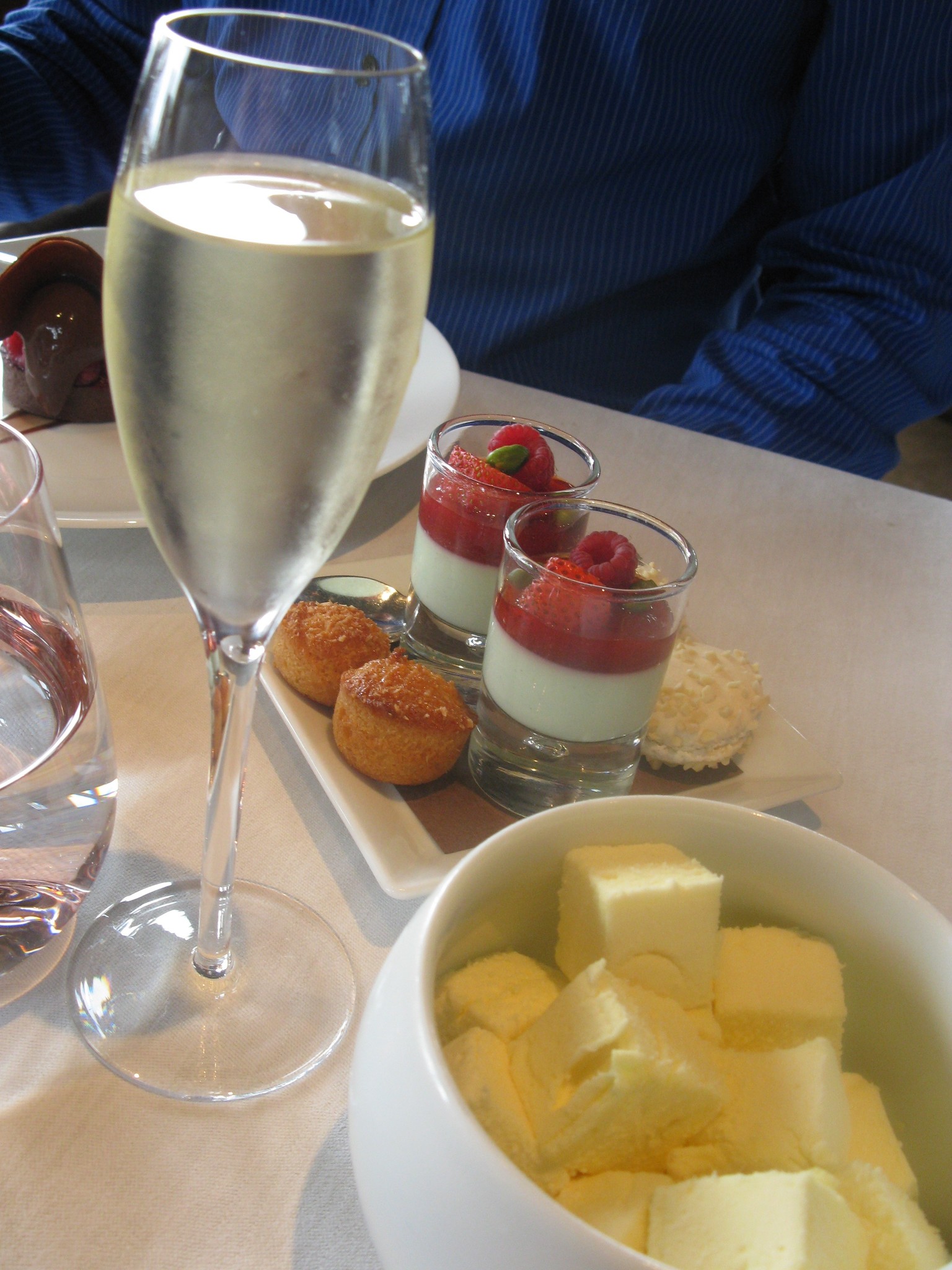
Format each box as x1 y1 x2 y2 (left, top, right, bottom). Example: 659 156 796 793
348 793 952 1269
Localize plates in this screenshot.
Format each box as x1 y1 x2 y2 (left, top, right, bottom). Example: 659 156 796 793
1 226 459 532
260 637 845 904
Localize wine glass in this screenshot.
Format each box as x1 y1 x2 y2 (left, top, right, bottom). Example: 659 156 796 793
66 7 436 1113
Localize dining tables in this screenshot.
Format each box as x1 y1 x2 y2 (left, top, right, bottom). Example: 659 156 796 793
0 225 951 1270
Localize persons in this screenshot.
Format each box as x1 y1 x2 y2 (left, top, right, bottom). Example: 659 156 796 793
2 0 952 480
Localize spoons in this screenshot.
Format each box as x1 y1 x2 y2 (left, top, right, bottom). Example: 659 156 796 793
293 574 415 641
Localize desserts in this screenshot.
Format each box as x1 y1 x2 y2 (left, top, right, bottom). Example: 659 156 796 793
436 842 952 1270
274 599 391 708
410 422 589 635
640 635 774 773
0 235 115 423
482 532 676 743
333 657 480 784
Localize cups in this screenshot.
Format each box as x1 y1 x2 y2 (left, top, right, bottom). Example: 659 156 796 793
400 411 601 707
468 496 701 816
0 412 119 1014
